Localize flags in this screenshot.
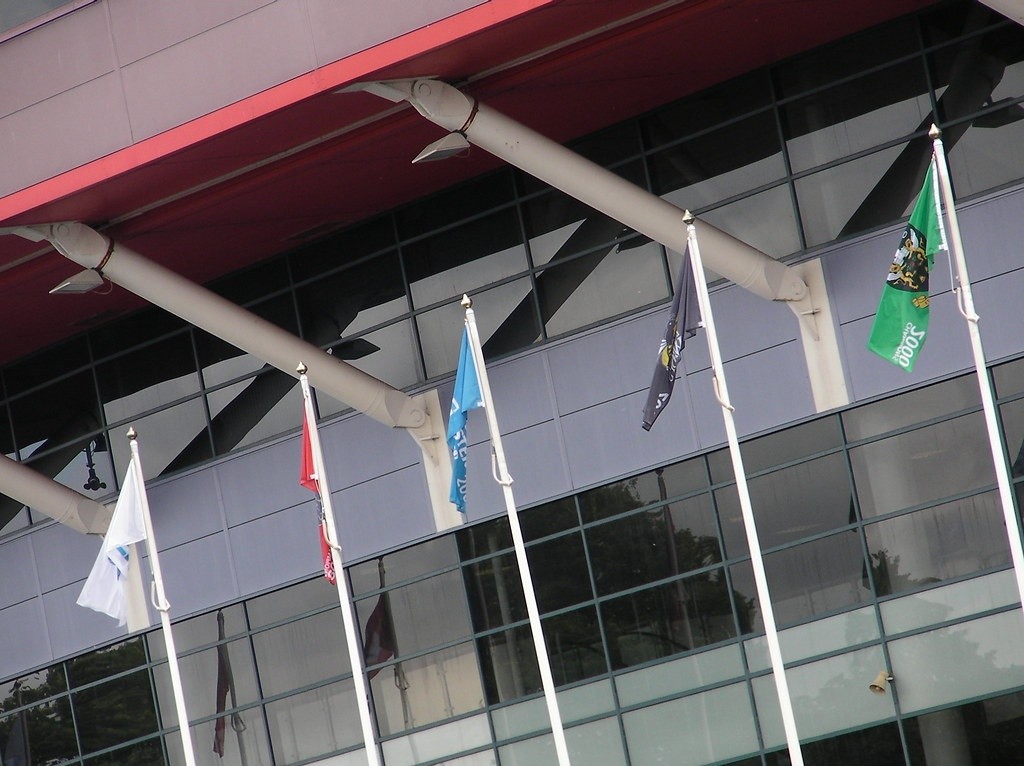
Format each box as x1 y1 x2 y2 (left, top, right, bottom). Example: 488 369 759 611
641 238 706 431
299 405 338 584
866 153 948 373
75 460 149 629
213 625 228 758
364 594 394 681
447 320 486 513
